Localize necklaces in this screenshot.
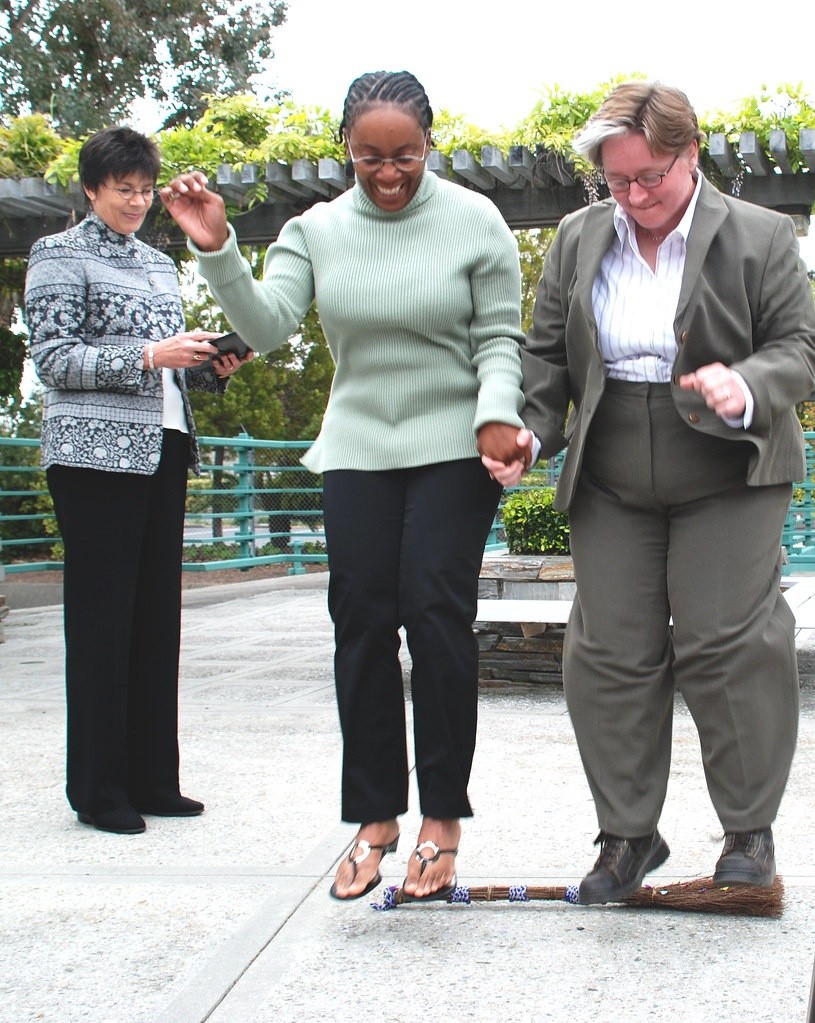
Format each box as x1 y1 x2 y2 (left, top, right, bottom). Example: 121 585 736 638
639 228 672 241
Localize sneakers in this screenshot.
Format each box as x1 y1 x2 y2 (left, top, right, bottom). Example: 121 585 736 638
578 826 671 905
714 826 776 886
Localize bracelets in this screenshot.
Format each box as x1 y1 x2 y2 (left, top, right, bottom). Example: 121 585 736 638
147 343 155 371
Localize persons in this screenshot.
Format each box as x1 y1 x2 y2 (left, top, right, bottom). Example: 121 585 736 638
23 126 254 835
159 71 532 902
480 82 815 905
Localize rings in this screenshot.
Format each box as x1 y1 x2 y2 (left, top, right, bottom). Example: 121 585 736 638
228 366 233 371
724 387 731 400
193 353 202 361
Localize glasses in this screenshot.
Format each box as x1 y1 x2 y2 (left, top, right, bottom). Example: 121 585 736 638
345 131 428 174
601 154 681 193
99 179 161 201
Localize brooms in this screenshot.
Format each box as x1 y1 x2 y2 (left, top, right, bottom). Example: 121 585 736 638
370 873 787 920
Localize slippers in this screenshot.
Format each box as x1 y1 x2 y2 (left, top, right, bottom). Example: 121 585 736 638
402 840 459 901
330 831 400 901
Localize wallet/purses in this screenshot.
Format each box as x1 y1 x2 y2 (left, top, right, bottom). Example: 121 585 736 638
193 331 250 372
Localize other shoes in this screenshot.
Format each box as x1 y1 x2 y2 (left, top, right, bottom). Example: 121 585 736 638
77 808 146 834
125 791 204 817
